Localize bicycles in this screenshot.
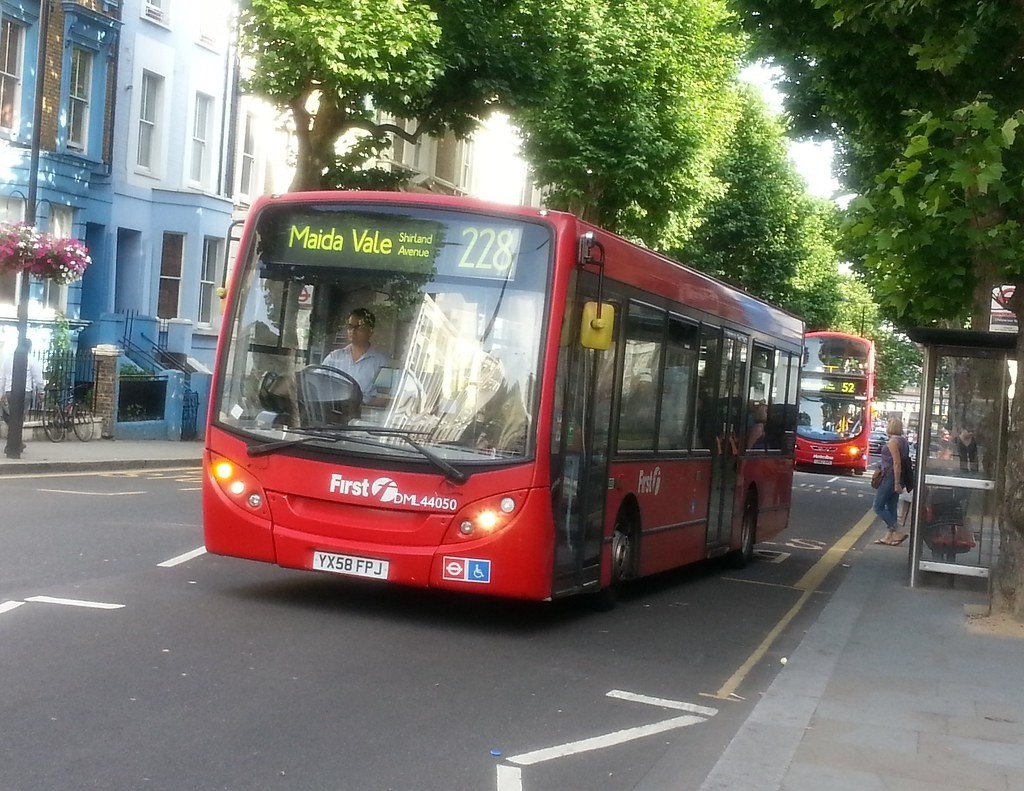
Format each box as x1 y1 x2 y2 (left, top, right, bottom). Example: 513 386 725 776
42 383 94 443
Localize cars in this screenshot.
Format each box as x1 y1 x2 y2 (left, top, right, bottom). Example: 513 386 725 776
869 426 918 454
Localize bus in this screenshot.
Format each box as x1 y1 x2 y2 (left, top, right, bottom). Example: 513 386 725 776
776 331 875 475
203 190 806 614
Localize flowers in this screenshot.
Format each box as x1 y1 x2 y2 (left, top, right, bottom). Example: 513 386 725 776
0 219 93 287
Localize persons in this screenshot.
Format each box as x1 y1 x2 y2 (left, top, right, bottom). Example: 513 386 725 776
949 422 981 518
872 417 914 545
312 308 395 418
698 378 766 448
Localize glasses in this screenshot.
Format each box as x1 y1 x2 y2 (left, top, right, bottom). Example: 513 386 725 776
346 323 369 331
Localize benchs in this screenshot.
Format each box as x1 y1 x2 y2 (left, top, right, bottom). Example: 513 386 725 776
923 486 976 588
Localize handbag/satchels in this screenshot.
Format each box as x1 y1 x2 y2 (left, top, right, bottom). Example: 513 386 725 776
871 465 883 489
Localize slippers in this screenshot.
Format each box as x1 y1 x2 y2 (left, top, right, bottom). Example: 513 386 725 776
892 535 909 545
876 539 890 545
